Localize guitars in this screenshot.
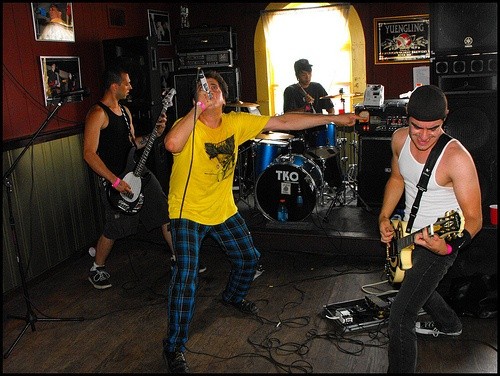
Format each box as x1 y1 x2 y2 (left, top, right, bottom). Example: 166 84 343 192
109 87 177 215
385 207 466 283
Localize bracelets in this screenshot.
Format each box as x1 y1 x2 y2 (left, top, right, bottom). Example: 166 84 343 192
112 177 121 187
446 243 453 255
306 105 310 111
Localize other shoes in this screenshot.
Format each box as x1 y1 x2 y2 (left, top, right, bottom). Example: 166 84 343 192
86 267 113 290
169 257 206 274
161 348 188 375
221 291 259 314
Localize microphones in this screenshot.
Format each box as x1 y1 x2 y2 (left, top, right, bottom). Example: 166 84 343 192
59 88 91 97
198 66 213 99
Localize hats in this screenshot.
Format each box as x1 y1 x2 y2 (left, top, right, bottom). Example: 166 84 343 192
294 59 313 71
407 85 448 121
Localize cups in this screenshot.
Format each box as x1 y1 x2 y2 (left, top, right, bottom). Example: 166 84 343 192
489 205 497 225
339 109 343 115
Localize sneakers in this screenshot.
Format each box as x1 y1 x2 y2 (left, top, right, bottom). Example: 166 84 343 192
415 319 462 338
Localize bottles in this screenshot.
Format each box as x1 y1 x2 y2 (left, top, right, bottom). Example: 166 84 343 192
278 199 288 225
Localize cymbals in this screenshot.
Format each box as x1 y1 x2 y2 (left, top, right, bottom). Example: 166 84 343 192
226 100 260 107
319 93 361 100
254 131 295 139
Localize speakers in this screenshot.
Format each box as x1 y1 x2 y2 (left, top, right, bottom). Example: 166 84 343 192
356 134 407 208
170 67 239 120
428 0 500 230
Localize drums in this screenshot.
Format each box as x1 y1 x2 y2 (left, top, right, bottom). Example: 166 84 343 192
304 122 338 160
246 140 290 183
254 154 324 223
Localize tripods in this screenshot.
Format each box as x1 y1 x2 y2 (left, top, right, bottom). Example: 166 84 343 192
1 96 87 359
323 127 370 222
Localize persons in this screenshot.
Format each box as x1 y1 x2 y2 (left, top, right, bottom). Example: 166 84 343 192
161 69 364 373
283 59 335 115
39 4 73 41
378 84 483 374
82 68 207 290
48 63 61 97
